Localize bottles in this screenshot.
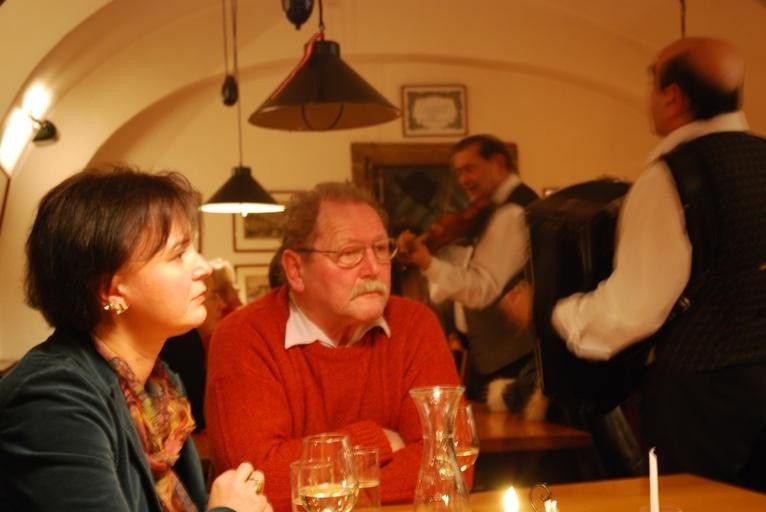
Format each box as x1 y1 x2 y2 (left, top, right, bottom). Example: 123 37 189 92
408 383 473 511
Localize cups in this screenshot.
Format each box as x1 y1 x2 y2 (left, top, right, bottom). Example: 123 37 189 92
449 405 480 472
290 432 382 511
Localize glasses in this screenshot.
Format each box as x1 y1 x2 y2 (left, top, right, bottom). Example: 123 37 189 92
294 238 399 268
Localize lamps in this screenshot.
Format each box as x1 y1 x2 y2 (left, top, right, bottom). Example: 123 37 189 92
28 112 58 148
194 0 289 218
246 0 404 134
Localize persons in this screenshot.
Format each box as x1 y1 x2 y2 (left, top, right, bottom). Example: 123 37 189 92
497 35 766 493
203 181 475 512
161 277 229 435
396 134 541 413
208 258 244 320
1 168 274 512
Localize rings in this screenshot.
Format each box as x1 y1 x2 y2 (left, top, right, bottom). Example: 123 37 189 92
247 478 261 492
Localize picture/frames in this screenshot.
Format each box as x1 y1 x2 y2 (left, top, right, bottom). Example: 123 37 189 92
402 82 470 137
233 264 273 309
232 192 294 252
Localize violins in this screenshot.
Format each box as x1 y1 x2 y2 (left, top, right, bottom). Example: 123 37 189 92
397 195 496 261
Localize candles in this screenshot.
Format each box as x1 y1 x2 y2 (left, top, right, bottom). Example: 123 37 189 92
649 446 661 512
